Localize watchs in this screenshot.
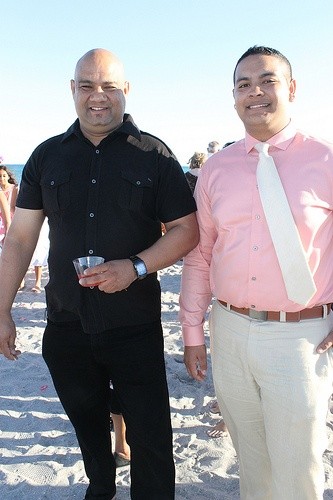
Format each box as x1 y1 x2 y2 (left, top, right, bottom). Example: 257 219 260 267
129 254 148 280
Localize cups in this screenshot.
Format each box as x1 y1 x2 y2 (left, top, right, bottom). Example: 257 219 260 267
72 256 104 287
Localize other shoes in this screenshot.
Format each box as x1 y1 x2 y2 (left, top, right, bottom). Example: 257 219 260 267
113 451 130 466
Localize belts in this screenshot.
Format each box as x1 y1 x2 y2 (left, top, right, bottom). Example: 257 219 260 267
219 299 333 322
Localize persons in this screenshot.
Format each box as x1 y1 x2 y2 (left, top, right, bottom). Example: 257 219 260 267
107 385 131 466
30 217 49 293
0 48 200 500
181 137 239 192
179 45 333 500
0 166 25 291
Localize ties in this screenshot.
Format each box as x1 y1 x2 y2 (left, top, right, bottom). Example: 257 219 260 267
252 140 317 307
